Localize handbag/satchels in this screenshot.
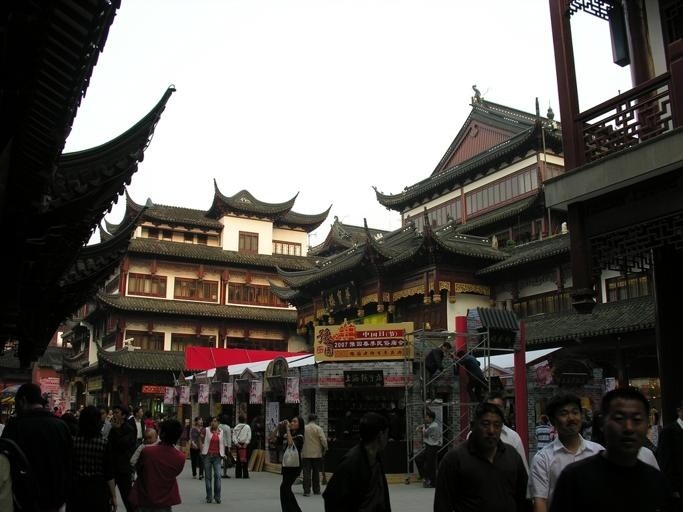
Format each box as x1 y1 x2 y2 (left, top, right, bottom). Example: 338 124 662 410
239 448 248 463
282 442 301 469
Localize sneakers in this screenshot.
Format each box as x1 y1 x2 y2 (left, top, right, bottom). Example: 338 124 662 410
426 399 443 404
199 475 204 480
221 475 230 479
207 498 221 504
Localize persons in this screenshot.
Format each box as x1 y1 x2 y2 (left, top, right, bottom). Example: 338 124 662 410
420 342 451 403
321 412 392 511
189 414 252 504
415 411 441 488
451 351 485 403
275 415 329 512
434 384 683 512
0 382 185 511
